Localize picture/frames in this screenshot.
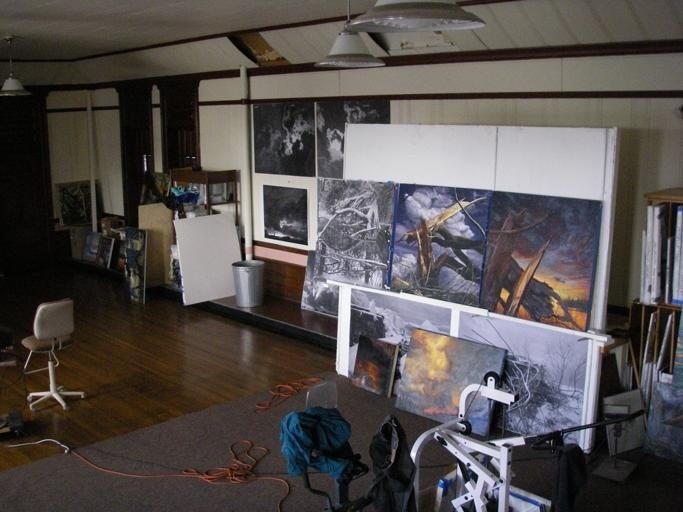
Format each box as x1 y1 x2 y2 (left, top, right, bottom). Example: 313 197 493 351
83 223 149 306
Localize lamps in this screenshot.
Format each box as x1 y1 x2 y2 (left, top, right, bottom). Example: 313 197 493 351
312 0 487 72
0 34 31 97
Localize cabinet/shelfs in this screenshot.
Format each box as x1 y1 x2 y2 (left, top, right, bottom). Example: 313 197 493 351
631 184 682 409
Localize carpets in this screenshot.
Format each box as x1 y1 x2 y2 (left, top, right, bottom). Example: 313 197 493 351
0 369 682 511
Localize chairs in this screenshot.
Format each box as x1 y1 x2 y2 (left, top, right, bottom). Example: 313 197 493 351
1 296 87 441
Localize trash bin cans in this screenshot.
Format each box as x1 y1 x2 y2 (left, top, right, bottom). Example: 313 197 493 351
232 260 265 308
